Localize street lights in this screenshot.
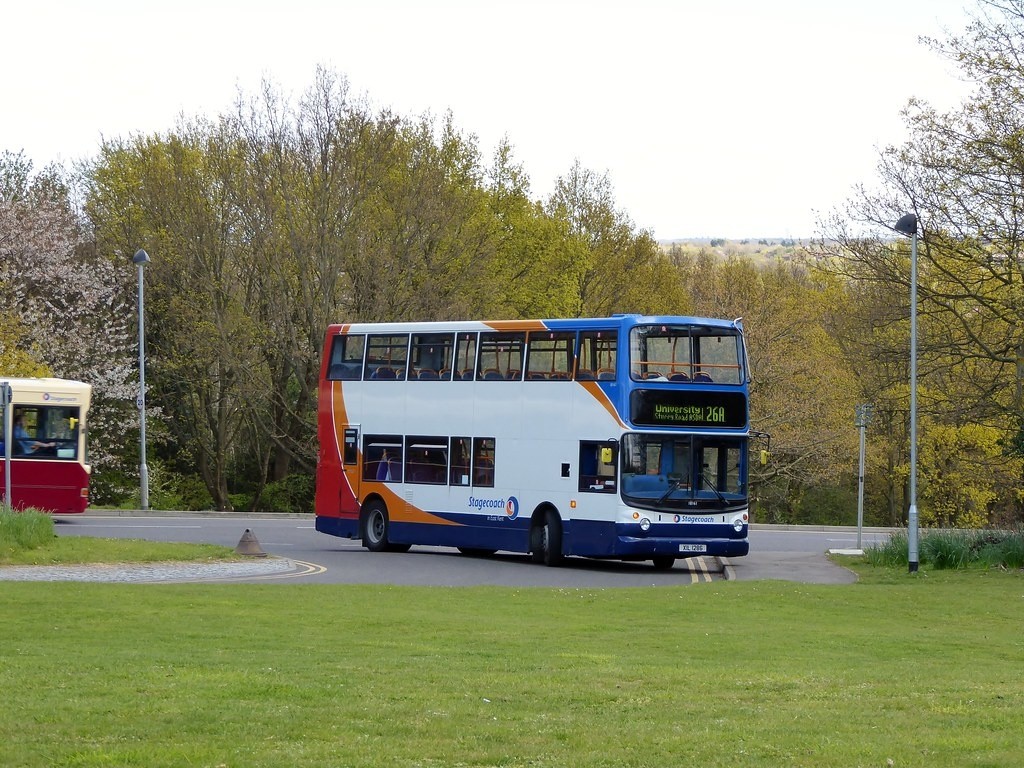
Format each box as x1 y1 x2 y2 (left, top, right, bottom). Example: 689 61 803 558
894 214 921 575
132 249 152 508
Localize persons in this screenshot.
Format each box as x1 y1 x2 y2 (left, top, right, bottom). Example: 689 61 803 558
14 414 55 454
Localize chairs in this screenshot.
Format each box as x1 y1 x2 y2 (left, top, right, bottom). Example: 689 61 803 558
597 368 615 380
643 372 663 378
377 452 493 485
483 369 504 380
505 369 573 380
693 372 713 382
578 369 595 379
632 369 644 379
667 372 690 381
462 369 482 379
330 364 462 380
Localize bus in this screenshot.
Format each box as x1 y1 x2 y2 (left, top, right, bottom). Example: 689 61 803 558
0 378 93 513
315 313 775 569
